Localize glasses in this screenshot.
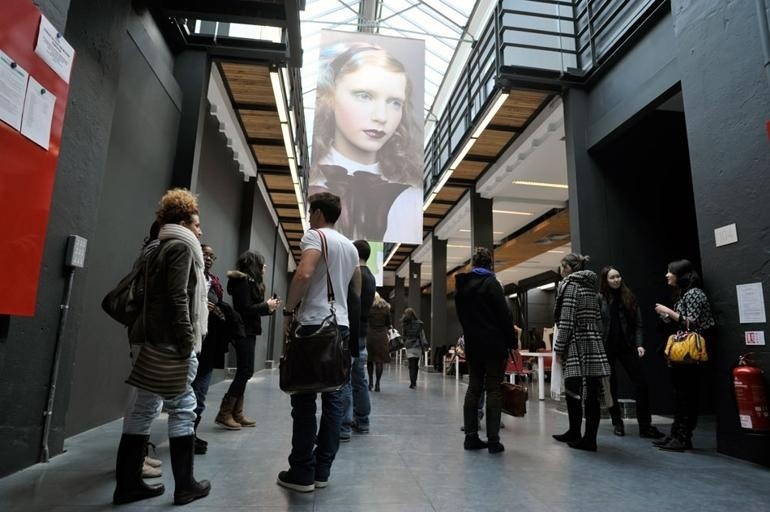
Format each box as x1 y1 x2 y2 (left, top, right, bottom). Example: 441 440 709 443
203 253 218 262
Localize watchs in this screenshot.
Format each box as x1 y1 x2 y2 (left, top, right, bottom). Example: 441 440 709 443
282 307 295 317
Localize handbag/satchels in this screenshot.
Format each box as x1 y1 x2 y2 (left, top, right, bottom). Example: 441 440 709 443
499 380 529 417
662 318 709 365
278 313 354 395
124 340 190 396
387 328 405 352
580 376 615 409
101 269 148 327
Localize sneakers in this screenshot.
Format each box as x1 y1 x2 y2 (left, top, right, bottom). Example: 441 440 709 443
315 475 329 487
352 422 369 434
313 425 351 443
277 470 315 493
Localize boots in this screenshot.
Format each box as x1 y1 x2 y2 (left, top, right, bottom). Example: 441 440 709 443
233 394 255 427
461 409 505 431
214 393 242 430
552 399 583 442
193 415 208 453
567 399 600 451
113 434 165 505
409 376 417 388
169 433 212 506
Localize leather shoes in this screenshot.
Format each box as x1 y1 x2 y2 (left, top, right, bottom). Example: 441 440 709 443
651 435 673 445
464 435 488 450
144 455 163 467
613 423 625 437
375 384 380 392
640 426 665 438
368 383 373 392
488 442 504 453
141 464 162 477
661 438 693 449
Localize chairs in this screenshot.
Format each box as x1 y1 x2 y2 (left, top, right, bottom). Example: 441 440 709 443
395 344 555 401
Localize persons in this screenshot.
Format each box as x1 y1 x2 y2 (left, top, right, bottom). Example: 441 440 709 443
215 251 282 431
188 244 224 456
276 191 364 492
112 189 211 506
338 240 376 442
454 248 513 453
369 290 546 392
306 43 424 244
552 254 612 451
597 265 666 439
653 259 718 450
121 218 163 478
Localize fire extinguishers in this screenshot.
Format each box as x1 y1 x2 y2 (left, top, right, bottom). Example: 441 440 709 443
733 351 769 431
